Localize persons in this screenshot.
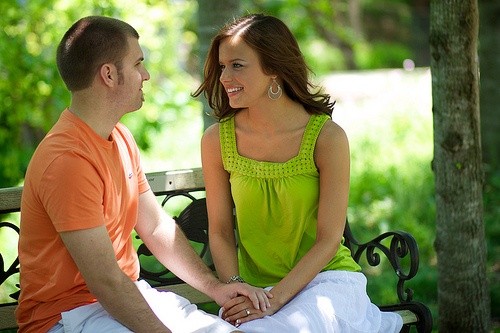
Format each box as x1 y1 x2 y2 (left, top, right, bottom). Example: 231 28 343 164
14 15 273 333
190 12 403 333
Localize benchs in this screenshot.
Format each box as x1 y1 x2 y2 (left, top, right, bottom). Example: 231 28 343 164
0 165 434 333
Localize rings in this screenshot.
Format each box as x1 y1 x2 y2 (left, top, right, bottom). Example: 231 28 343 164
246 309 250 315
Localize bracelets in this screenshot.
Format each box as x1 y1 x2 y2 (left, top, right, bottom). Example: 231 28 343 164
226 275 243 284
